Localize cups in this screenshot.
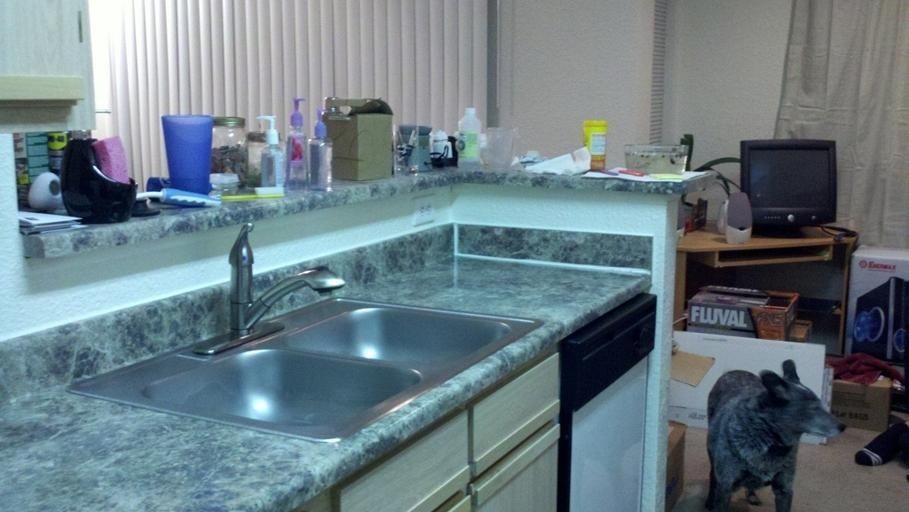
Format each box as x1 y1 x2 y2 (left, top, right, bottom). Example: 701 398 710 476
584 121 607 169
392 135 420 175
484 126 516 169
161 115 211 196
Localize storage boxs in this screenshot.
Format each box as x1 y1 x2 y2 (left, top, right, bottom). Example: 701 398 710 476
665 420 687 512
685 283 799 342
832 372 892 432
319 95 397 185
845 245 908 375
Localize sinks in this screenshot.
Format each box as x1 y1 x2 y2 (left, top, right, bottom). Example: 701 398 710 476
64 349 422 443
285 296 547 364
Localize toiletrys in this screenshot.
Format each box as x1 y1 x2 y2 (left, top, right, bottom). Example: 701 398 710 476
581 119 609 170
255 115 284 188
284 99 309 190
309 107 333 191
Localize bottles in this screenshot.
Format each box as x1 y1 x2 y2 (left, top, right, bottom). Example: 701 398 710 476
429 130 452 158
247 132 282 187
211 116 248 189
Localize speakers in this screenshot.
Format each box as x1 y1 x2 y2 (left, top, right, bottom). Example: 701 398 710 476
726 192 753 243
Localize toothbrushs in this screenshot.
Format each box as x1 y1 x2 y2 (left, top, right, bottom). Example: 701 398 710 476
135 186 222 208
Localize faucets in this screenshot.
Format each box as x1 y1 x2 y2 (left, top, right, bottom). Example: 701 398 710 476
229 223 346 337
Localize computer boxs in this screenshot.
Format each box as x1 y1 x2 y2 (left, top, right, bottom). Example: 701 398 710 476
852 277 904 364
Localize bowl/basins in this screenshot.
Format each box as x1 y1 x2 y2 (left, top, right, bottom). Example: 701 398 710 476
624 144 689 176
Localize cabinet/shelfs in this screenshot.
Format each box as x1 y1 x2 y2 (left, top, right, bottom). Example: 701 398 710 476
331 351 565 512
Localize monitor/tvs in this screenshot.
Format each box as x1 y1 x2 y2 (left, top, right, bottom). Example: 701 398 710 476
741 139 836 237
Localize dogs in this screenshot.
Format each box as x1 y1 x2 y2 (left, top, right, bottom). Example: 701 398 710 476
704 359 846 512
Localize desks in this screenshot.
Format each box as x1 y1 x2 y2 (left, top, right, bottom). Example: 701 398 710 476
674 219 860 355
